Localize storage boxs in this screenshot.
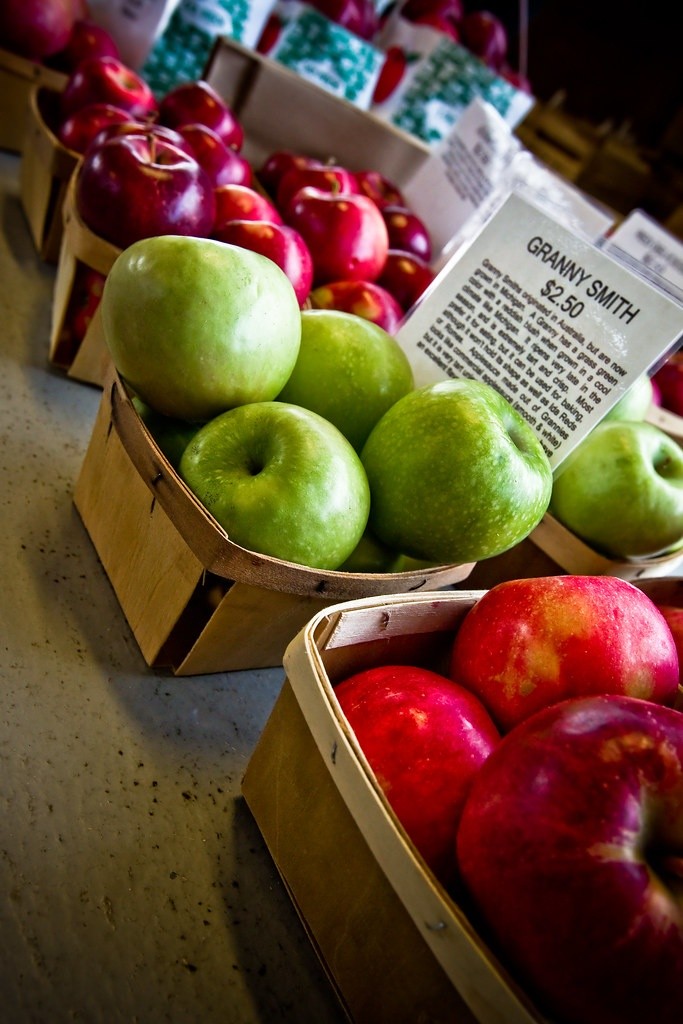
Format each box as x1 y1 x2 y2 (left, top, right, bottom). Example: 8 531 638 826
28 86 683 1024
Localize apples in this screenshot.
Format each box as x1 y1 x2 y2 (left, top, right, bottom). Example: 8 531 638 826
327 0 530 95
554 368 683 555
100 235 554 575
335 576 682 1024
0 0 436 348
